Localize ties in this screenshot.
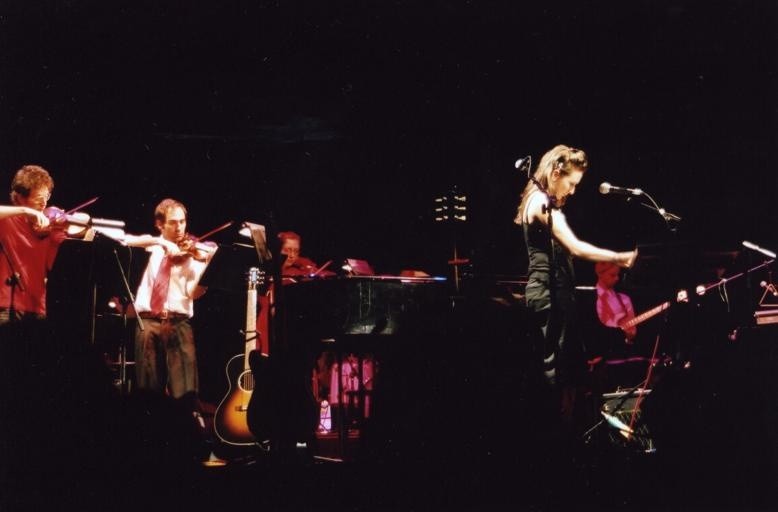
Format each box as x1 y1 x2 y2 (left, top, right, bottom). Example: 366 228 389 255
150 249 177 316
599 292 616 324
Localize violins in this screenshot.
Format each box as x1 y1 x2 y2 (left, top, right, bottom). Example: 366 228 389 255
164 237 217 260
281 257 336 278
33 206 128 236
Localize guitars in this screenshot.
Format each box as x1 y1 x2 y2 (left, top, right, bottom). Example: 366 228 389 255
213 265 300 446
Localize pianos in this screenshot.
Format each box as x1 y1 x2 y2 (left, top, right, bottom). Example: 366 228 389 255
614 240 735 296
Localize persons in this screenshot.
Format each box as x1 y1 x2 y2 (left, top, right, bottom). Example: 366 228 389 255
123 193 221 398
275 231 306 274
685 239 738 318
515 144 639 387
0 164 81 324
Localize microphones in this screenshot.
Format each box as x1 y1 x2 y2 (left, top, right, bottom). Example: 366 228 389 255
598 182 643 196
760 281 776 296
515 156 530 171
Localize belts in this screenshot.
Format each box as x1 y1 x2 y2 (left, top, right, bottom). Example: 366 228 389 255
135 311 191 322
